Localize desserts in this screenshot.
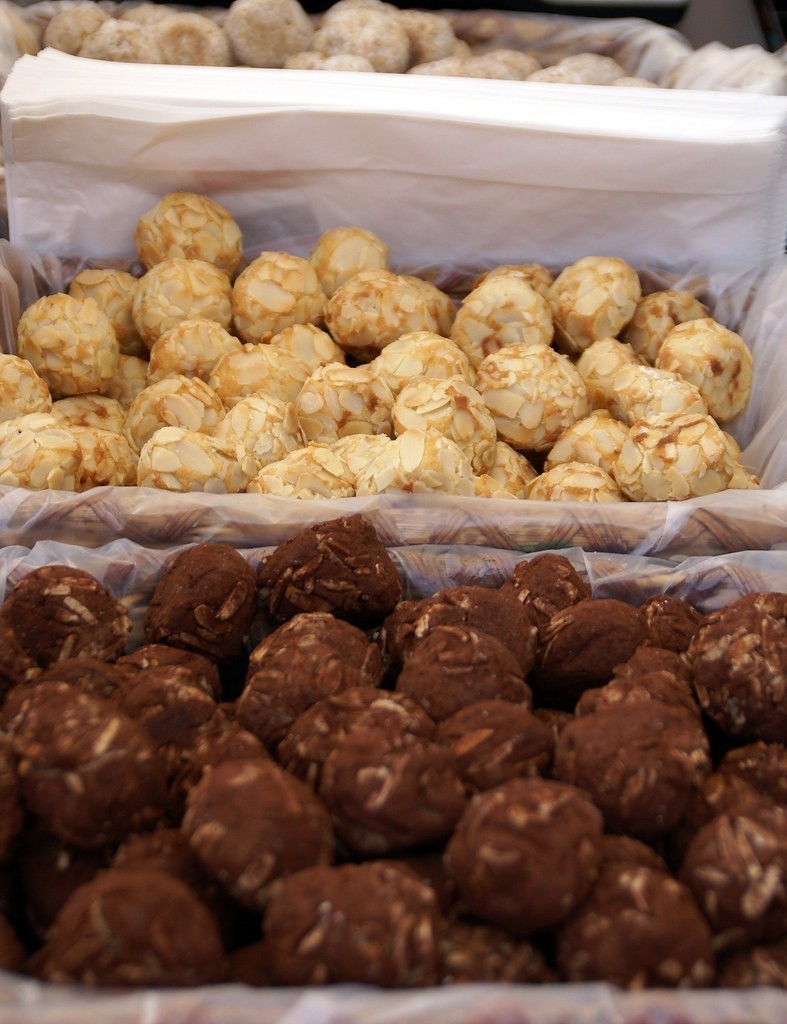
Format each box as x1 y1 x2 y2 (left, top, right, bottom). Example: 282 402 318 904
0 511 785 1001
0 192 755 504
0 0 663 90
